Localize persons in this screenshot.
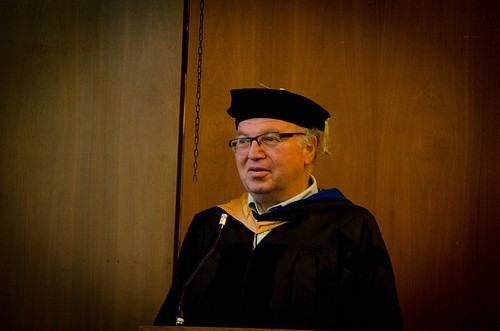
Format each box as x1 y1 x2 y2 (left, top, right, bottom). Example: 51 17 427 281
153 83 403 331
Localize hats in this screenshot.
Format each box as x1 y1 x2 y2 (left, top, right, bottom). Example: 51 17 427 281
226 88 331 131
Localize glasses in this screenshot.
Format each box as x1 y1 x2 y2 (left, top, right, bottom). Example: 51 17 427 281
228 132 318 153
